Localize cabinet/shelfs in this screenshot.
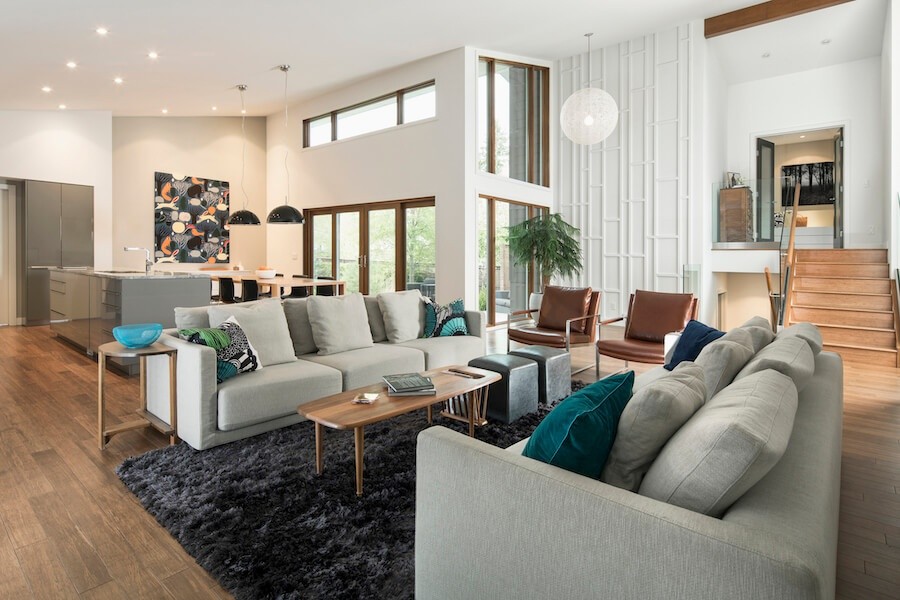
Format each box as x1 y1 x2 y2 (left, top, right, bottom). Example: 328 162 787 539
719 188 753 243
50 271 211 330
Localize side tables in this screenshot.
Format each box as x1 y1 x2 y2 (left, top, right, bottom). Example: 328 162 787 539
98 340 178 449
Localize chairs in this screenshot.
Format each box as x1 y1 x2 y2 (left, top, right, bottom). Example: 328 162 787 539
507 291 602 376
210 273 336 303
595 294 700 380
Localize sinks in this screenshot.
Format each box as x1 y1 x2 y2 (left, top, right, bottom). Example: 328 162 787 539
96 271 146 273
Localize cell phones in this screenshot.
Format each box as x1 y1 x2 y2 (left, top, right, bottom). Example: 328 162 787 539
354 393 379 403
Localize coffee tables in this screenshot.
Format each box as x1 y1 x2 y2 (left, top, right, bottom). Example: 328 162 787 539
296 365 502 497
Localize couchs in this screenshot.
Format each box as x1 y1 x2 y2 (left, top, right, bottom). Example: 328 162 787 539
146 295 486 450
414 331 843 600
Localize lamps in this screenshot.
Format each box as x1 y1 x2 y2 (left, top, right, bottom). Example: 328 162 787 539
228 65 304 225
559 32 619 145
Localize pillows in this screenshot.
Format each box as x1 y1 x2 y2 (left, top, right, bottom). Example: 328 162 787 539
420 296 469 338
534 284 592 333
177 315 264 384
626 290 694 343
306 292 374 356
521 319 775 494
376 289 425 343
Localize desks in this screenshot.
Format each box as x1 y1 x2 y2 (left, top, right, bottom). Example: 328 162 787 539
211 275 346 299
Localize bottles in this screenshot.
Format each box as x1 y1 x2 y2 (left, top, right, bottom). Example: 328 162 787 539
233 262 243 271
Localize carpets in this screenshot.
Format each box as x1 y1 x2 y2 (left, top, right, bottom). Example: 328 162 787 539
117 380 590 600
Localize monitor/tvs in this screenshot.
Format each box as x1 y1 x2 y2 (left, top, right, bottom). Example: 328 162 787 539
781 162 834 207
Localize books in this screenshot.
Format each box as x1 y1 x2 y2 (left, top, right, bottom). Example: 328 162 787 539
382 373 436 396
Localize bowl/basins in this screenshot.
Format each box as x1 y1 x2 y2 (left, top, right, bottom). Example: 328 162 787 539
255 270 276 278
112 323 162 348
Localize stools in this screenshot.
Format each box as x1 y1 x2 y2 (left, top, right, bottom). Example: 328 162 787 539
468 345 572 424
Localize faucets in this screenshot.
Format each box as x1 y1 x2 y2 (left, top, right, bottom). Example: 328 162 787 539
123 245 154 273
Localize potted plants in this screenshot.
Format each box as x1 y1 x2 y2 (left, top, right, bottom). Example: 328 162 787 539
501 211 584 324
